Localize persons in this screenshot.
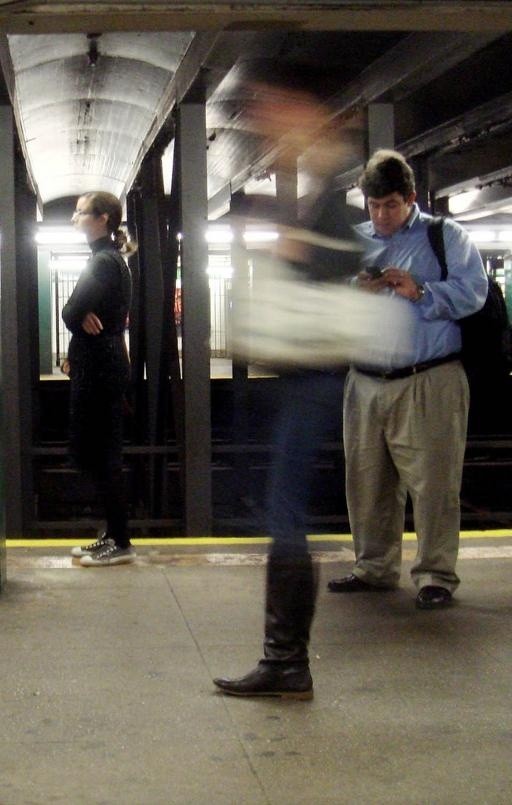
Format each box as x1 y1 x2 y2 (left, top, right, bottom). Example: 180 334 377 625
209 69 413 699
323 147 494 616
58 189 137 568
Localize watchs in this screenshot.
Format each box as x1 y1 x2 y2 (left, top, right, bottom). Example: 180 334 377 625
410 284 424 304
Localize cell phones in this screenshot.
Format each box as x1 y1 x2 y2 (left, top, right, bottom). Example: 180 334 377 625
366 266 382 279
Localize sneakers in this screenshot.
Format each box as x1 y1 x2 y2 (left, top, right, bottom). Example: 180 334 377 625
70 539 137 567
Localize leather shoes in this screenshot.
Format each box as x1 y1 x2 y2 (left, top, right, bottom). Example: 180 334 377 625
415 584 452 609
212 663 314 700
327 572 399 592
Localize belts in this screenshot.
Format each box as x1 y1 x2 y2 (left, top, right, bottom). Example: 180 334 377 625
352 352 464 379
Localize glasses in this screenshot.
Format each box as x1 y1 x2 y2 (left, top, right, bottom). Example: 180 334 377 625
72 210 102 218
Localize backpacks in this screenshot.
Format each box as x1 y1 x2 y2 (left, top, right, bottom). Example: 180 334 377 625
426 217 509 346
